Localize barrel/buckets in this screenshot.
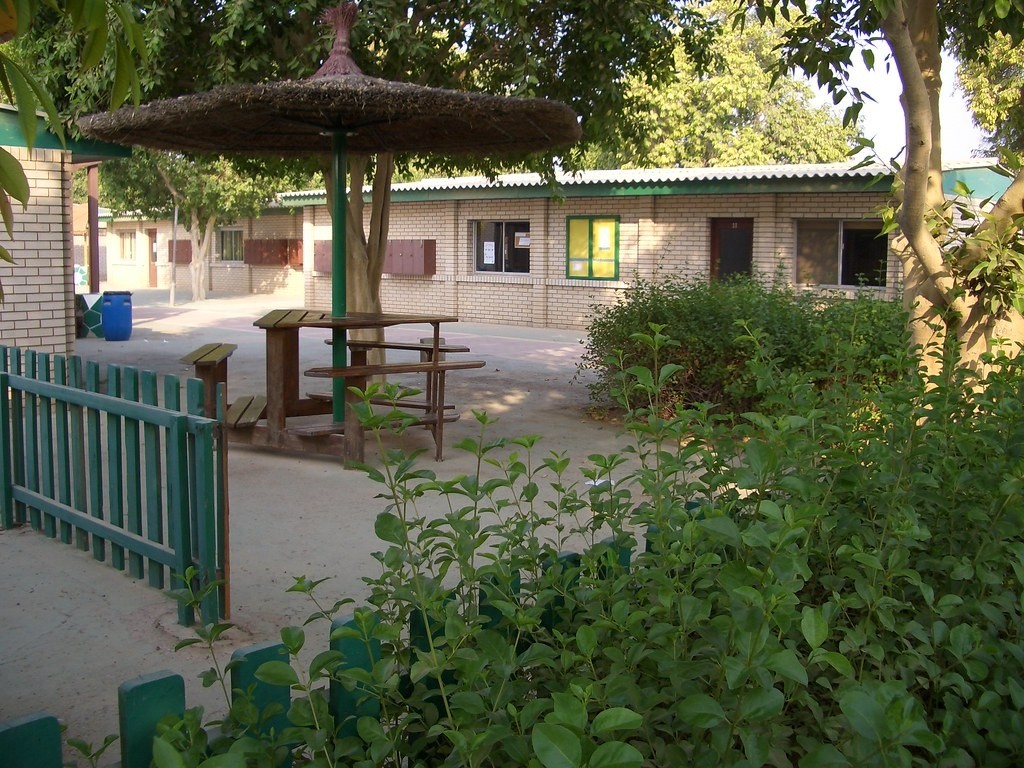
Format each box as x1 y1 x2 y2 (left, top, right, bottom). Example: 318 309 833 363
102 291 132 341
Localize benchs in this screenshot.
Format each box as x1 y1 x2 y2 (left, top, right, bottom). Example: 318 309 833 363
325 339 470 433
177 342 238 419
303 360 486 467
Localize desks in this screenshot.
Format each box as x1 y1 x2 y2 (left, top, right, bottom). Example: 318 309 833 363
253 310 459 469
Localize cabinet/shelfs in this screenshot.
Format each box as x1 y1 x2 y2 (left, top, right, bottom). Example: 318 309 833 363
313 240 332 273
381 239 436 275
243 239 303 265
168 239 200 264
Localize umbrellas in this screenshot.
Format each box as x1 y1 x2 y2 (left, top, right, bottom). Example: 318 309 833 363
76 6 584 422
